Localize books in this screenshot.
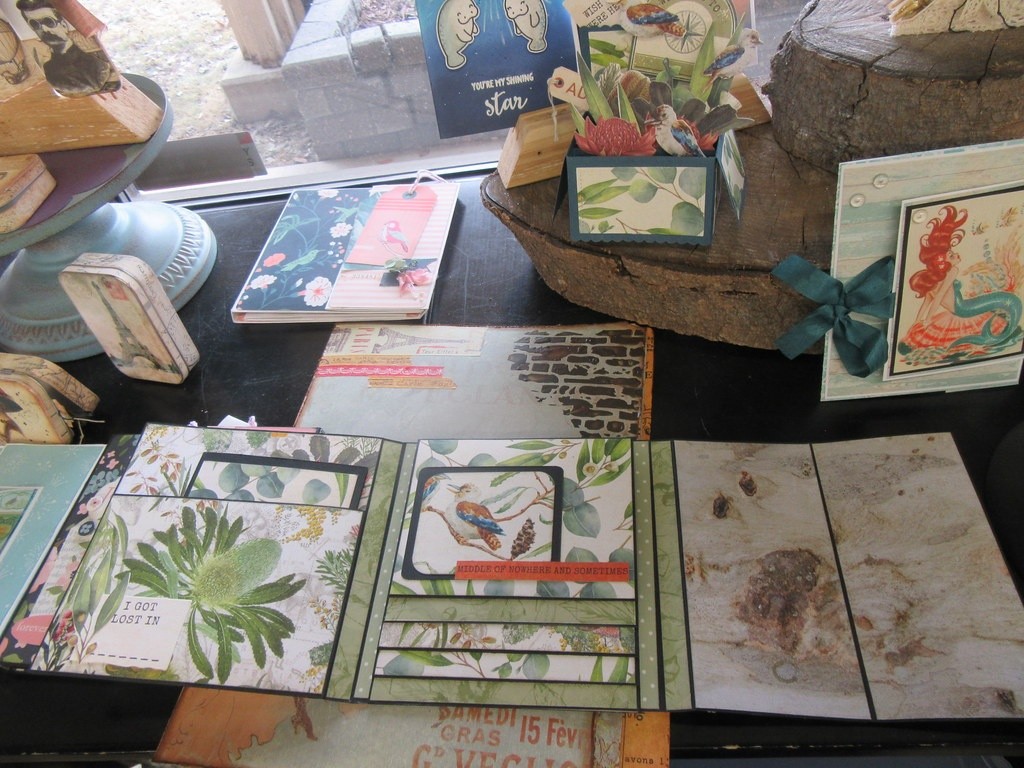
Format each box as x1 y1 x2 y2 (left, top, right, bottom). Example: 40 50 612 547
229 168 461 322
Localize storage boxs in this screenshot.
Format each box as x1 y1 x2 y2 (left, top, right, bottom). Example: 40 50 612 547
552 111 747 246
0 154 200 446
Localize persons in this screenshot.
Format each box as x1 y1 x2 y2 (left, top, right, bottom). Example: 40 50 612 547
18 0 108 95
